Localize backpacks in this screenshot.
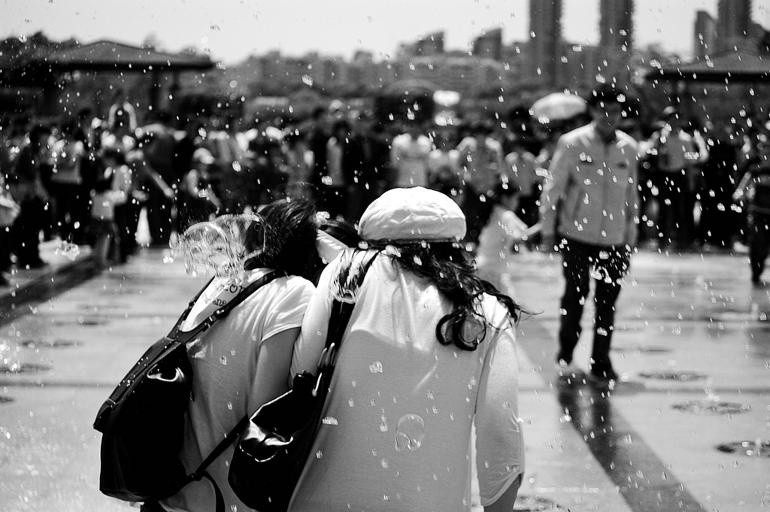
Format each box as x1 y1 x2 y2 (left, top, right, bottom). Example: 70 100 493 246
93 269 284 505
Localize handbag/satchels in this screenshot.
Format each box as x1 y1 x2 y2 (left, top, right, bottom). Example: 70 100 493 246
226 365 333 510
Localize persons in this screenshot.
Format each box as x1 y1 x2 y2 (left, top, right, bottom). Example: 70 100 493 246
538 85 638 383
1 81 768 285
288 186 526 512
142 196 316 510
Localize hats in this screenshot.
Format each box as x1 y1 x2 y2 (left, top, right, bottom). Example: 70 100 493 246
358 186 468 242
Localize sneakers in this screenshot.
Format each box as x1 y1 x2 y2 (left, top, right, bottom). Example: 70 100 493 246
555 344 573 364
751 276 765 287
592 357 616 380
